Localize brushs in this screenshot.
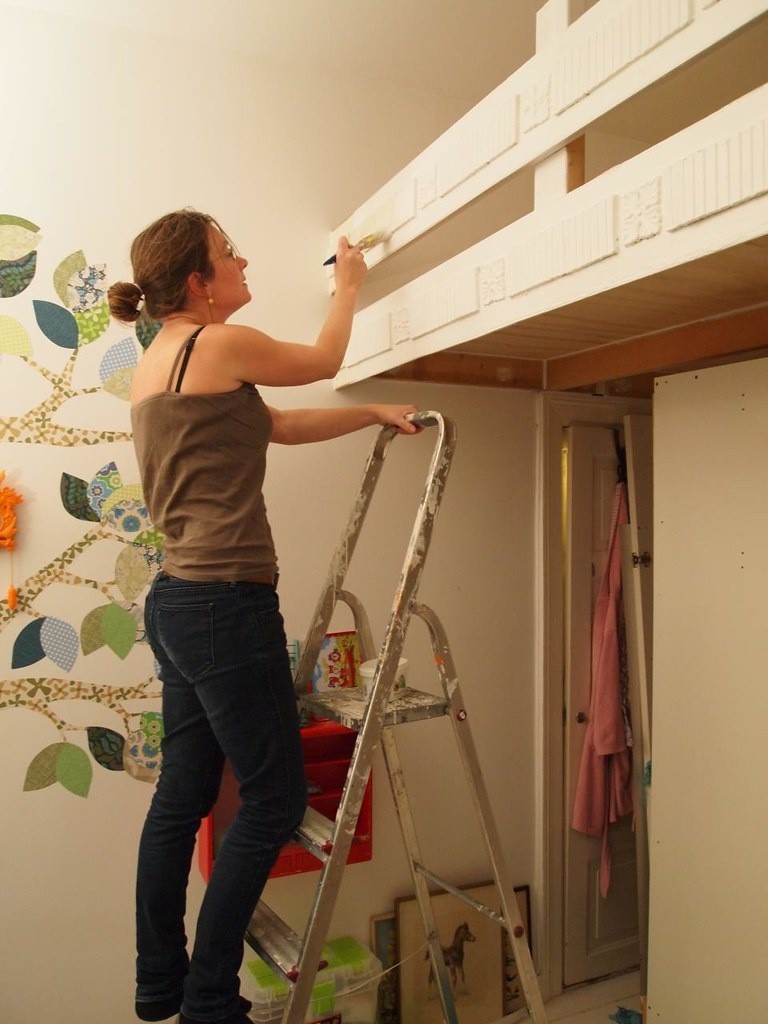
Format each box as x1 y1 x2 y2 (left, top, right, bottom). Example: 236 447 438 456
322 229 389 268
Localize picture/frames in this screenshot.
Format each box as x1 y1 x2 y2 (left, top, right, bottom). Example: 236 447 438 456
370 879 535 1024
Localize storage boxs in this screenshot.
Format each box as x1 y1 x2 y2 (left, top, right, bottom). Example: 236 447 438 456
229 933 387 1024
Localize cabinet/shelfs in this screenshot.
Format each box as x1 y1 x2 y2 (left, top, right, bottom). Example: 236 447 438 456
196 716 372 883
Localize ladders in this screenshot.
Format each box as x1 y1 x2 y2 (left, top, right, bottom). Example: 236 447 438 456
241 410 554 1024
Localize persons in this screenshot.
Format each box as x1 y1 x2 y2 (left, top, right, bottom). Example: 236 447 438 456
109 210 422 1023
